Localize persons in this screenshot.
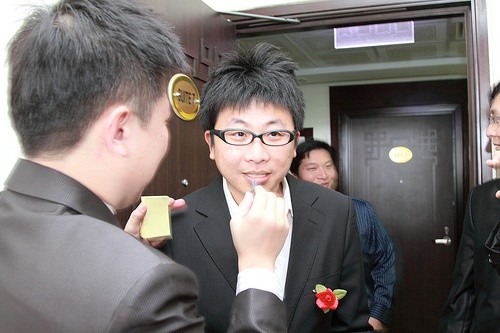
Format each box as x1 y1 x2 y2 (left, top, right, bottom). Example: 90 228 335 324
0 0 295 333
290 141 397 333
432 82 500 333
154 41 374 333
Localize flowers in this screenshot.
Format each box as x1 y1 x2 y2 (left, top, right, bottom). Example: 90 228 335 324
312 284 347 313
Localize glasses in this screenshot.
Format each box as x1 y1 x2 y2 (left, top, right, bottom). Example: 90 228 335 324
487 115 500 125
211 128 297 146
